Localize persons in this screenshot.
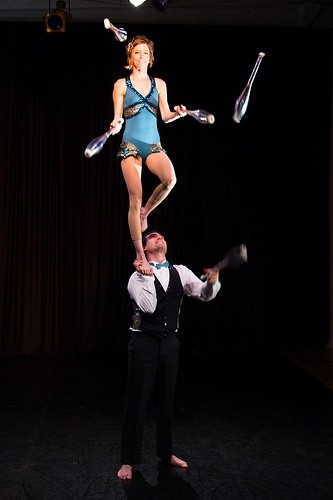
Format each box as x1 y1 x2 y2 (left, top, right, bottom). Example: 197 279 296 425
110 35 187 276
117 231 223 479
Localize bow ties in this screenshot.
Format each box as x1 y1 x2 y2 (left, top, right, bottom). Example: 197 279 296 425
155 261 169 270
